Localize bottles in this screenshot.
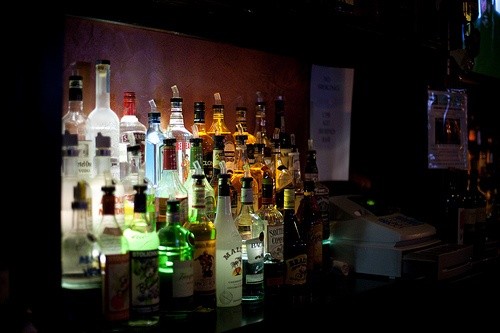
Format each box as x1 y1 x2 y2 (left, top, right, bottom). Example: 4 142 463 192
62 57 329 328
441 170 488 250
